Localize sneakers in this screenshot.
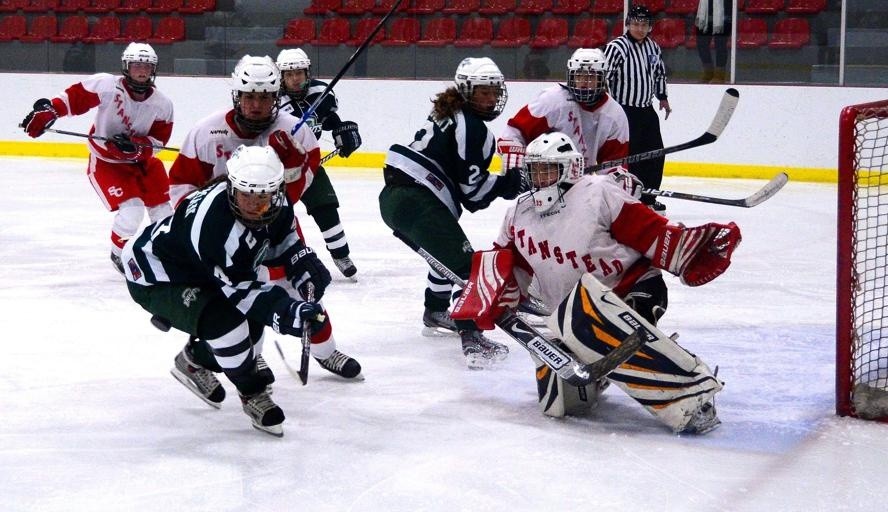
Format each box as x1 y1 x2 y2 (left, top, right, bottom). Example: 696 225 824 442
423 307 458 331
315 348 361 379
333 257 357 277
174 351 225 404
653 202 666 211
111 252 125 274
236 389 285 426
686 404 721 434
460 329 509 359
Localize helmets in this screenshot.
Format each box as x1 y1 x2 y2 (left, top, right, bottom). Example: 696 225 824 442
226 143 287 229
121 42 158 91
566 47 609 103
274 48 311 96
624 4 651 27
230 53 281 132
454 54 508 121
522 131 584 213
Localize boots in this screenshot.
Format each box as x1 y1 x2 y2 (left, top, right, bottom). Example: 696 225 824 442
709 66 725 84
699 61 714 82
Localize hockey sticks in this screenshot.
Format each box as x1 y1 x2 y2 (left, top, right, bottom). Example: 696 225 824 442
392 229 648 387
643 171 789 209
583 88 740 173
273 282 318 388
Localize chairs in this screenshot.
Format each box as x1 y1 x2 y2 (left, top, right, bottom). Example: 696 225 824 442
491 17 531 50
305 0 340 15
591 0 626 16
114 0 152 15
685 17 768 50
87 16 119 44
382 17 420 49
338 0 374 16
21 16 58 43
50 16 91 41
48 0 80 14
516 0 554 15
768 16 811 49
785 0 827 14
23 0 48 12
81 0 111 13
665 0 698 16
146 17 186 46
529 18 566 49
0 16 26 42
454 18 492 49
346 18 385 49
647 19 686 49
0 1 30 13
744 0 785 14
146 0 183 14
276 18 316 48
303 2 341 17
311 18 351 48
479 0 518 16
611 20 626 43
566 18 608 48
442 1 480 16
178 0 217 15
633 0 666 17
553 0 591 17
417 17 456 47
371 0 409 16
113 17 151 46
408 0 445 17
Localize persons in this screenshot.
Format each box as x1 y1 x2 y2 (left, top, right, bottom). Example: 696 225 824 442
169 55 363 380
695 0 732 84
18 42 174 278
122 144 331 438
379 56 531 372
449 133 742 436
276 49 362 283
498 48 643 201
604 7 671 216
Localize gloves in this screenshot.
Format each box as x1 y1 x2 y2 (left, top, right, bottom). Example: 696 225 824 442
331 121 362 158
598 160 643 200
266 129 309 178
20 97 56 138
112 131 136 154
269 297 325 338
284 248 332 302
504 166 527 200
